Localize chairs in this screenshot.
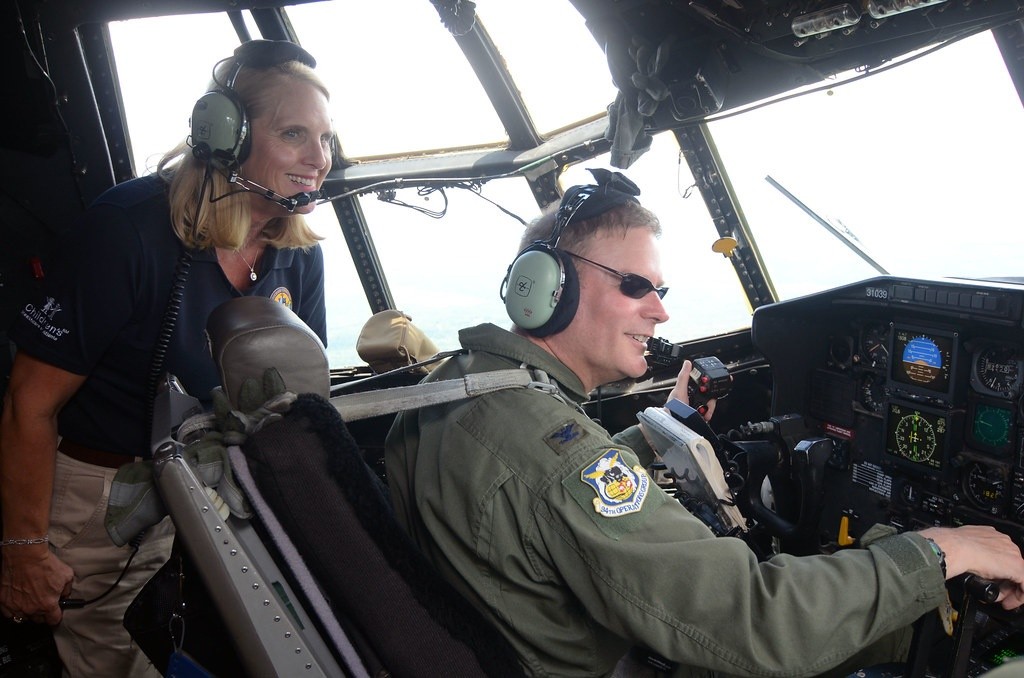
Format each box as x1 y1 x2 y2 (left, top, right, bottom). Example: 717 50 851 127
105 297 531 678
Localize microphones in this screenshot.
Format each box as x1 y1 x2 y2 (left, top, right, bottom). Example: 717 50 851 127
192 143 321 213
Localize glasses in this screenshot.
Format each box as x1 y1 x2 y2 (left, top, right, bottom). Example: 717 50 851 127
564 250 669 300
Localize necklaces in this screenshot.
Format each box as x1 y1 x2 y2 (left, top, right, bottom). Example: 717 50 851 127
231 246 261 282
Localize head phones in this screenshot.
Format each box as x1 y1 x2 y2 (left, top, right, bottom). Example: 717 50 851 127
187 40 317 169
501 167 641 338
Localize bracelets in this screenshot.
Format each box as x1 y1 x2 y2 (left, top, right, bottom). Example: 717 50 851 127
0 533 50 547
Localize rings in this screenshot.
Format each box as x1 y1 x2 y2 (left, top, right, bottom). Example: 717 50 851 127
13 616 23 625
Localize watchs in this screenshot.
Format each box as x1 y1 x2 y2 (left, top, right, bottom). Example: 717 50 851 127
919 534 947 585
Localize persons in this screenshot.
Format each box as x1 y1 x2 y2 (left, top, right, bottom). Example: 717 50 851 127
382 190 1023 677
0 44 335 678
